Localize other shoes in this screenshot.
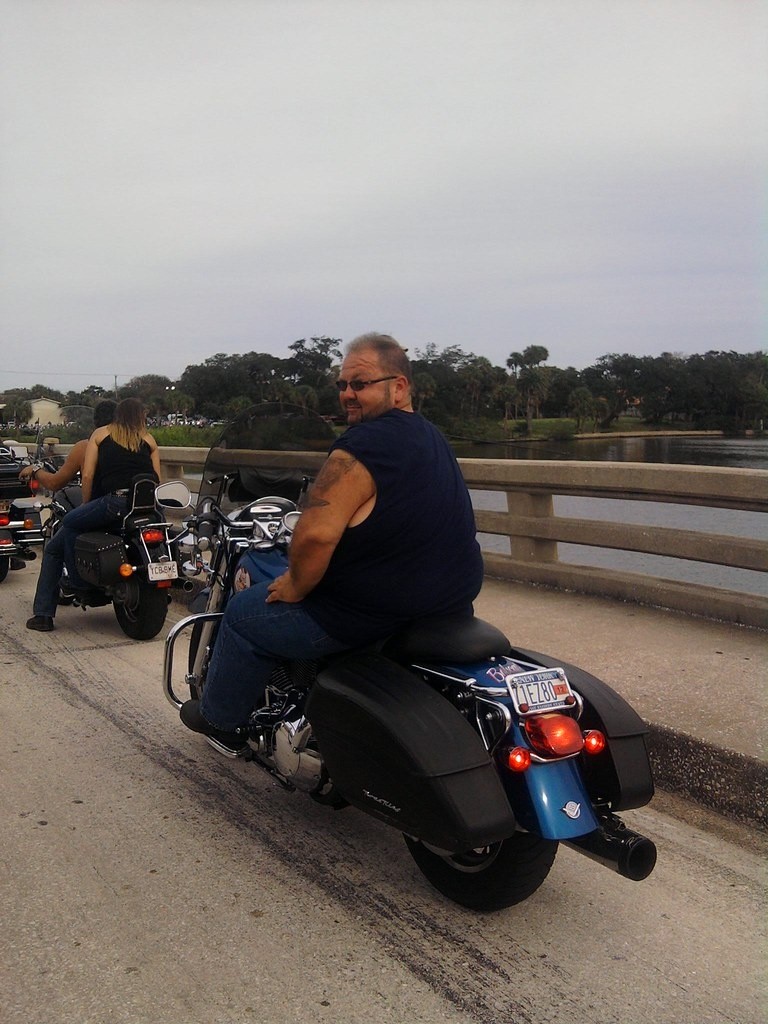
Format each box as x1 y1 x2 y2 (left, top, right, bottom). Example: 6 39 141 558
12 548 37 561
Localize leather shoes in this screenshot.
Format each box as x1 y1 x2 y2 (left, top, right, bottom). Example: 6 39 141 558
26 615 54 631
180 698 249 744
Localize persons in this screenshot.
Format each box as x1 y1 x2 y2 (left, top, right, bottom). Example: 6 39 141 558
59 398 161 599
18 400 120 632
177 330 484 762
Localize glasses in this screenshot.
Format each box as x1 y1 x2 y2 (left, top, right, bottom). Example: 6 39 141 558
336 376 397 391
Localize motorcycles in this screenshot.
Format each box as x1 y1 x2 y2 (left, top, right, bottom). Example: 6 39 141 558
27 403 198 640
1 489 36 585
135 399 663 912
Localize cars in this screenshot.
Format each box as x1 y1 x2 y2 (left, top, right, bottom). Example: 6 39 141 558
0 417 232 496
